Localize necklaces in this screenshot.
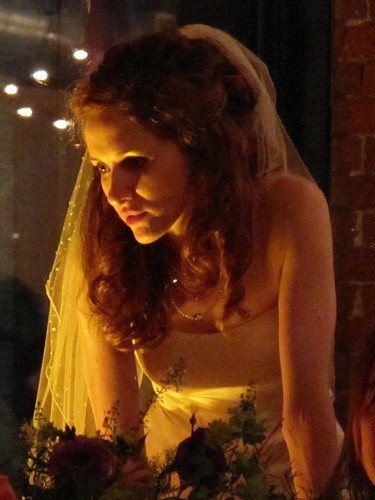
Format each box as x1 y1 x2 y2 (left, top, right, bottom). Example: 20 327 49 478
169 288 229 322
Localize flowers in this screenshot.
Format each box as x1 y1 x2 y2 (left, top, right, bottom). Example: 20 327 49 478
3 357 375 500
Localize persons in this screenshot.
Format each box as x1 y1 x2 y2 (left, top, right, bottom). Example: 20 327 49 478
26 23 345 500
